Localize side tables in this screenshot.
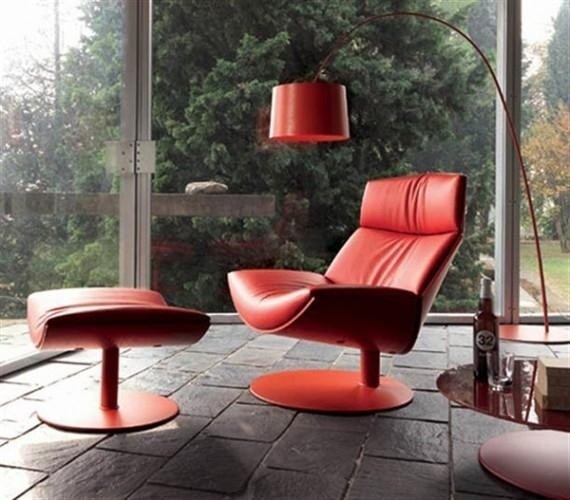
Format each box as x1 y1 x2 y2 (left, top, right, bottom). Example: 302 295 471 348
434 354 570 500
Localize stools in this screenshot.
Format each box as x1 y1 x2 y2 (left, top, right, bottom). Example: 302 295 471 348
20 287 211 436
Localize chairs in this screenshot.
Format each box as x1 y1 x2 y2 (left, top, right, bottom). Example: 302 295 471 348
225 171 469 416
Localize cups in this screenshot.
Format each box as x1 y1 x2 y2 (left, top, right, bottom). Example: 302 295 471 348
485 351 515 393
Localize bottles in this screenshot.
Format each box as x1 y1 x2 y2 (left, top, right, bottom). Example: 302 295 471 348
471 275 500 384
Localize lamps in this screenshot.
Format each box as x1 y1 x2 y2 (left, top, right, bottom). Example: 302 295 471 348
266 11 570 346
